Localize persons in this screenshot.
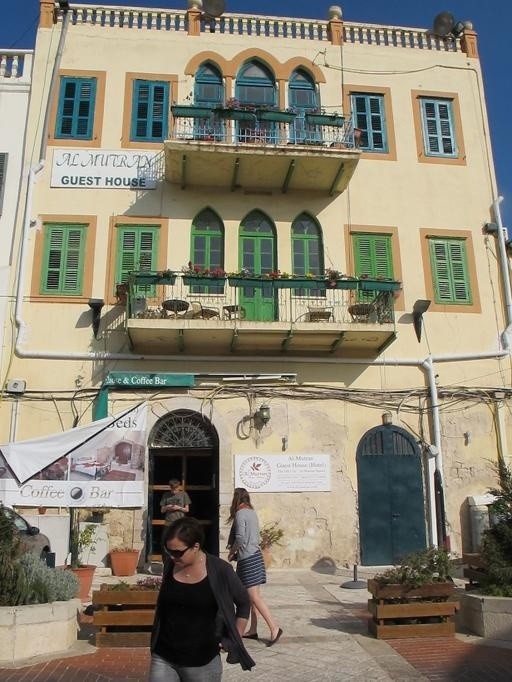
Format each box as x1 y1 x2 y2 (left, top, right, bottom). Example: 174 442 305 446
158 478 192 549
227 487 283 646
147 516 250 680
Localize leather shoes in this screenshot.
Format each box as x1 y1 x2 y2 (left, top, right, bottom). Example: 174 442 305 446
267 627 282 647
242 633 258 639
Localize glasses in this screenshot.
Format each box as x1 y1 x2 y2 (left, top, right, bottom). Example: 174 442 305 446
165 545 191 559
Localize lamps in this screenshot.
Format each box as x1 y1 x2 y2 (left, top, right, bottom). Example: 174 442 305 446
259 401 272 427
383 409 395 427
413 300 431 342
88 298 105 338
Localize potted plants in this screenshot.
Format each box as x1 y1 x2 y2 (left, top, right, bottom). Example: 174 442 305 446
260 519 285 572
57 524 163 651
367 539 454 640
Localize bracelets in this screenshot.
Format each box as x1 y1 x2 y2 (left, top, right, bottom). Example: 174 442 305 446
178 507 182 511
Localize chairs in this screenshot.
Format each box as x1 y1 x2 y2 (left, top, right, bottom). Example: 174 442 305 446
132 301 390 323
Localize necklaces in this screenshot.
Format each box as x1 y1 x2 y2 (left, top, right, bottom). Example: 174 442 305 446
181 555 204 579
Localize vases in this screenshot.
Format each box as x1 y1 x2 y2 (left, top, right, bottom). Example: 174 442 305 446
170 104 345 127
134 276 401 291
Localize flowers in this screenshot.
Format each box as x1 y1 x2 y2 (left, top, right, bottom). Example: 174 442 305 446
157 262 385 287
220 94 329 116
